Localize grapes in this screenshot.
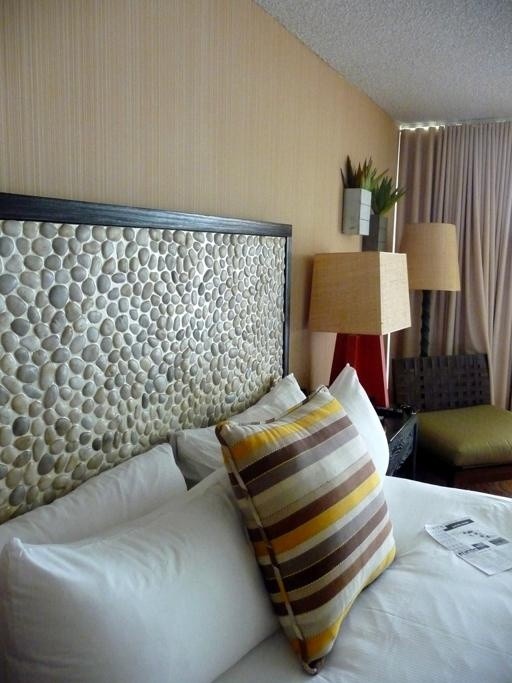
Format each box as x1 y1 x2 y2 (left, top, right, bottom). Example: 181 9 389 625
374 403 421 479
371 399 420 481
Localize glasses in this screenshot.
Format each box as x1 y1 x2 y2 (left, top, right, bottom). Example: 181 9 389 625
398 221 462 358
307 250 412 337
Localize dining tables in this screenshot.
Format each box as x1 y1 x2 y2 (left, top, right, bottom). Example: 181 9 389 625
362 176 411 252
340 151 390 237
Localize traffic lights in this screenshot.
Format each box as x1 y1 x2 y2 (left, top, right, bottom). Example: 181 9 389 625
0 465 281 683
327 363 390 486
1 442 189 550
175 374 308 483
214 385 396 677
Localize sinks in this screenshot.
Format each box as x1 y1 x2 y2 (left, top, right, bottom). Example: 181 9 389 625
392 352 512 488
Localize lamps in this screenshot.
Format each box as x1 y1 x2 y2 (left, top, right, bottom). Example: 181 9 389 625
391 351 512 487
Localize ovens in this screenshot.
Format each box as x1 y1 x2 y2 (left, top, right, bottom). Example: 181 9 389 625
0 189 511 682
